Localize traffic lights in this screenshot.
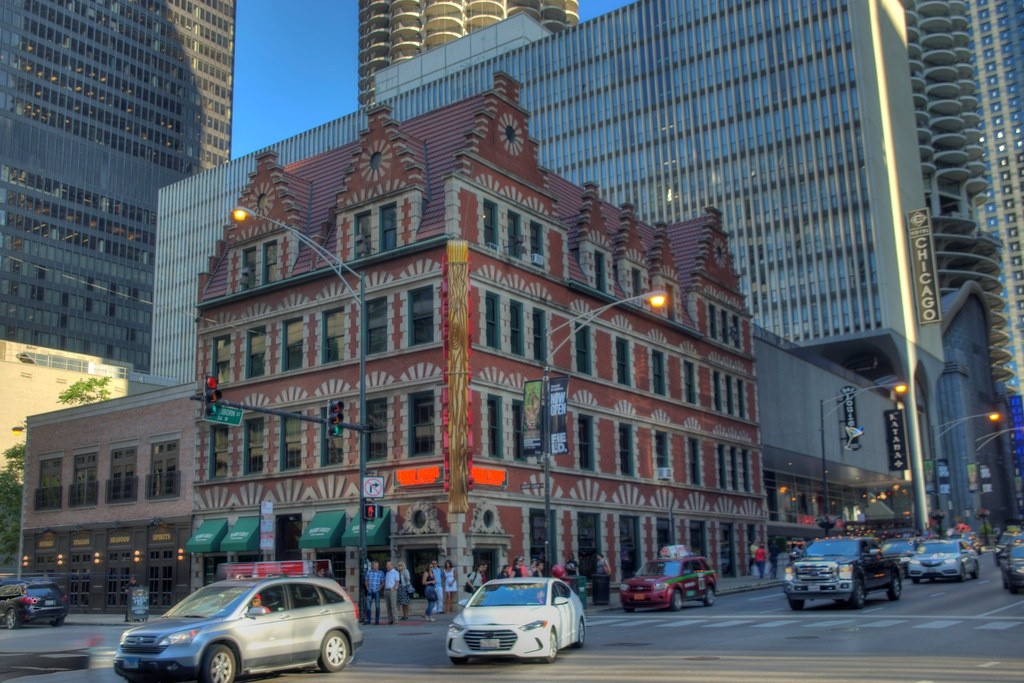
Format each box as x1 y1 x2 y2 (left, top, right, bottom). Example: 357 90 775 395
203 376 223 417
329 400 344 437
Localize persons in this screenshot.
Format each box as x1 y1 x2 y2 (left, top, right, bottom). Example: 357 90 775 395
316 567 324 577
748 540 767 579
496 557 543 579
466 562 487 596
384 561 411 625
768 540 781 579
597 552 611 575
123 576 140 622
362 561 385 625
252 594 270 613
564 553 583 575
421 559 458 622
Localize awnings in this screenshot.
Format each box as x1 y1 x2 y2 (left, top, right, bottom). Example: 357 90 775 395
299 510 346 548
186 519 228 552
220 516 260 551
342 506 391 546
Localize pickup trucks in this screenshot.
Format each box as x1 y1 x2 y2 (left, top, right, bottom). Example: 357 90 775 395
783 536 905 609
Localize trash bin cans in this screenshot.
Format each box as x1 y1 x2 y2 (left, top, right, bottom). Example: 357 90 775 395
592 573 612 605
570 576 588 610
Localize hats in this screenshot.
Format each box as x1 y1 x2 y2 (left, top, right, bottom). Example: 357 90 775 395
255 594 261 600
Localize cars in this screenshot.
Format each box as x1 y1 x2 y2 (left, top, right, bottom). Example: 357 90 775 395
879 538 927 573
907 540 980 584
445 577 587 665
992 531 1024 594
0 577 70 628
619 555 719 613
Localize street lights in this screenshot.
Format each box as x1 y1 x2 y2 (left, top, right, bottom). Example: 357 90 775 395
932 411 1001 536
231 206 368 624
818 381 907 516
541 289 669 577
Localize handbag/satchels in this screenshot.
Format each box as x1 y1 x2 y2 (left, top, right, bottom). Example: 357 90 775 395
406 584 415 593
464 570 478 593
603 559 611 574
427 582 439 602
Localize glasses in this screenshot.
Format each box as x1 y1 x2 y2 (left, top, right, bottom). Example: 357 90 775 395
429 566 434 568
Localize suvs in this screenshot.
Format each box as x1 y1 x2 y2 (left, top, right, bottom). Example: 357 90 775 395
113 571 365 683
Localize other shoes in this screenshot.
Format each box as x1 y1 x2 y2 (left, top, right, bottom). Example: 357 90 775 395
374 622 379 625
363 622 371 625
385 622 398 625
422 614 437 622
399 617 408 621
